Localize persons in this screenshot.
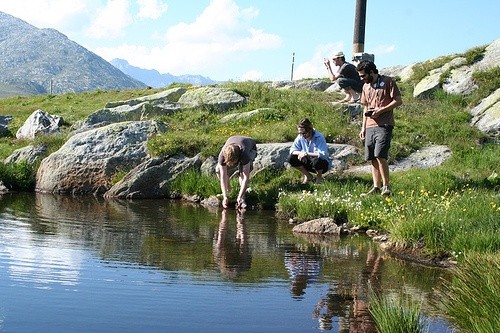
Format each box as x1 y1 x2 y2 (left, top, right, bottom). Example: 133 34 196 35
214 136 257 210
324 52 364 104
285 118 332 184
356 60 402 196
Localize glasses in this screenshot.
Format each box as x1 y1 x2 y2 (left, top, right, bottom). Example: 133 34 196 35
359 73 368 78
297 129 310 135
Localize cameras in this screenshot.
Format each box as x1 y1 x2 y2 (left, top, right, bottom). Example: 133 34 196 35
364 107 374 116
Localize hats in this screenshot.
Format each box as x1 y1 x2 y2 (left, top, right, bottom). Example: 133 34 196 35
331 52 345 60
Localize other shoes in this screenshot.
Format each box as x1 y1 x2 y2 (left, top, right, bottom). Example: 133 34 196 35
349 98 361 104
360 187 381 197
381 184 392 195
339 98 350 103
